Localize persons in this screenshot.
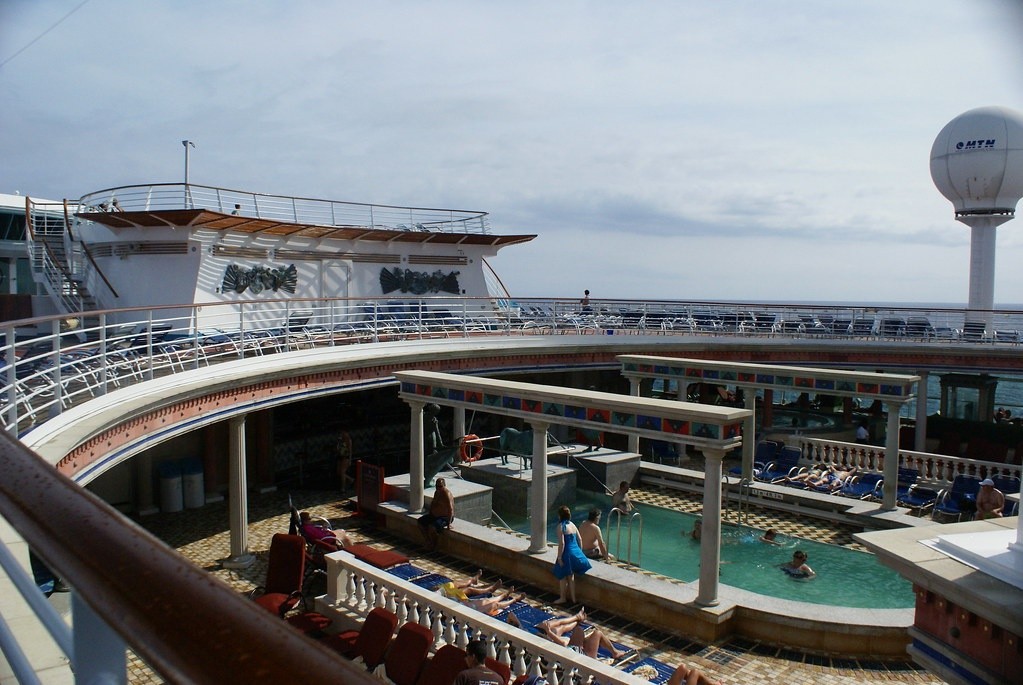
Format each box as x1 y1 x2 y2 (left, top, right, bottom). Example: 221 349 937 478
612 481 634 515
418 478 454 551
79 197 125 213
855 422 869 446
453 640 504 685
759 529 783 548
232 204 242 217
432 568 526 616
579 508 616 560
996 406 1023 425
974 479 1005 520
553 506 592 605
727 394 746 402
681 519 702 537
667 664 723 685
537 606 625 659
782 550 815 576
336 431 355 496
792 415 798 427
300 512 353 546
784 462 856 490
579 290 591 316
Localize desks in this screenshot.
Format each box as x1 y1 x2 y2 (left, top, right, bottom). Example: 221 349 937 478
1005 492 1020 516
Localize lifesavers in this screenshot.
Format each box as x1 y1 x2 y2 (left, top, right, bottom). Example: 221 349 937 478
460 434 483 462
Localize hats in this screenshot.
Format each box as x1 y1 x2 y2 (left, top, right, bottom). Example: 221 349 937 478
979 479 994 486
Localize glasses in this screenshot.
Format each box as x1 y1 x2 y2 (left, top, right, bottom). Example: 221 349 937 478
435 484 445 487
464 653 472 657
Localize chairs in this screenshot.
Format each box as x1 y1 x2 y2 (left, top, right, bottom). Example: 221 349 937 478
650 439 1021 523
0 301 1023 428
396 222 411 232
248 514 677 685
413 222 443 233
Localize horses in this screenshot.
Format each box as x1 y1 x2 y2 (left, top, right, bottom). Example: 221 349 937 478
500 428 551 470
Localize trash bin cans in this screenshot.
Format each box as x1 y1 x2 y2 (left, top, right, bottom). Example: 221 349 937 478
184 471 204 509
160 475 184 513
